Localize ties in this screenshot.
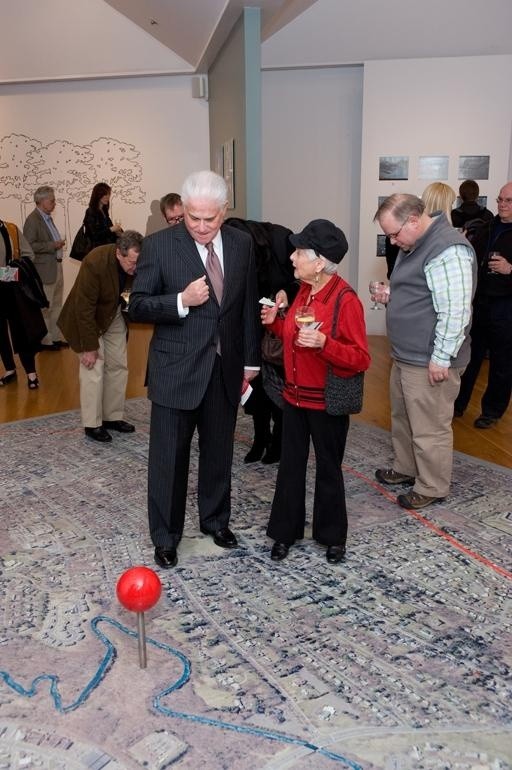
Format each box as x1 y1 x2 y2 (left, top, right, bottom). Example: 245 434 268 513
204 241 224 357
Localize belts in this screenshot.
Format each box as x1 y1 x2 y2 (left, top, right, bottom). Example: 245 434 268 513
56 258 63 263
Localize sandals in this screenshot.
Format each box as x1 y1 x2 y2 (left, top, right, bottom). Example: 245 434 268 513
0 371 17 386
27 375 39 390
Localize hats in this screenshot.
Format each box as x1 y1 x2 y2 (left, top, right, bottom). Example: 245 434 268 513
287 217 350 265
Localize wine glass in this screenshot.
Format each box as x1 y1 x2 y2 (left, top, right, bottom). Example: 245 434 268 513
369 280 384 310
293 306 315 348
114 219 122 236
487 250 500 274
122 287 133 313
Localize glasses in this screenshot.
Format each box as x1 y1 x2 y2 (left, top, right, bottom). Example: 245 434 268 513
495 197 512 203
166 216 185 224
386 215 410 240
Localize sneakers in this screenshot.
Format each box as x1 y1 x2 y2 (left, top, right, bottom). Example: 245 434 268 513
397 486 448 510
375 467 416 486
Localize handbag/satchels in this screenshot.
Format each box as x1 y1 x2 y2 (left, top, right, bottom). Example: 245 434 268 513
260 333 287 412
68 227 97 263
323 369 365 417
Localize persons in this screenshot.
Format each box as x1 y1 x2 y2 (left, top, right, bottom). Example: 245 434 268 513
224 217 301 465
419 179 512 429
55 230 145 442
159 193 185 228
370 193 478 510
0 218 39 390
128 172 262 569
260 218 372 564
83 182 129 342
23 184 69 351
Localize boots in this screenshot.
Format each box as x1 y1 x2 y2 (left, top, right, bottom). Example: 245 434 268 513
242 412 285 466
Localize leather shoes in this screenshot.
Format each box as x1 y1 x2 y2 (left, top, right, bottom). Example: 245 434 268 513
153 546 179 569
84 426 113 443
326 544 346 563
473 414 503 429
270 539 290 562
102 419 136 434
199 524 240 548
